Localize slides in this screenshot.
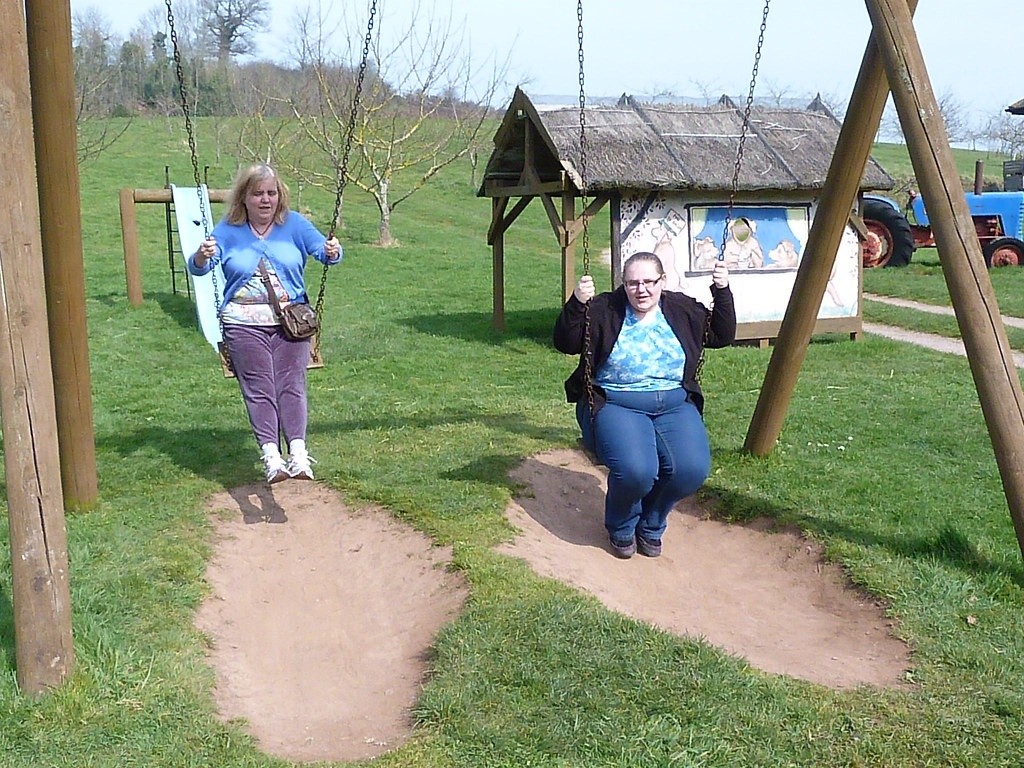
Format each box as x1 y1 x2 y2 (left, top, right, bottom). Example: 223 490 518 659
167 183 228 353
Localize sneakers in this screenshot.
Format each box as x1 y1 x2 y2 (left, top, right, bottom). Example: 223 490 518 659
261 451 290 484
287 448 318 480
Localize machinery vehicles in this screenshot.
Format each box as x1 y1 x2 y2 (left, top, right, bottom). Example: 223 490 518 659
862 158 1024 269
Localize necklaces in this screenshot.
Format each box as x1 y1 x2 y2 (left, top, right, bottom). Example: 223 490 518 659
251 217 275 239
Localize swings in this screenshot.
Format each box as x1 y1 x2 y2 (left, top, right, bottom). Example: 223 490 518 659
164 0 377 379
576 1 772 468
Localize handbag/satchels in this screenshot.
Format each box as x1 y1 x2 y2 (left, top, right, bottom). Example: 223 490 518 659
279 302 318 341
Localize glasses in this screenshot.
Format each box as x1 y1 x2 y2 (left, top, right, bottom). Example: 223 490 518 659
623 272 664 288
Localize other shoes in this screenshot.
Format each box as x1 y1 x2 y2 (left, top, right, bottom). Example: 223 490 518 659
636 532 663 557
607 533 635 559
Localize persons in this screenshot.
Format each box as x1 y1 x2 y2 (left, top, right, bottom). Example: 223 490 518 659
188 162 343 482
553 252 737 559
